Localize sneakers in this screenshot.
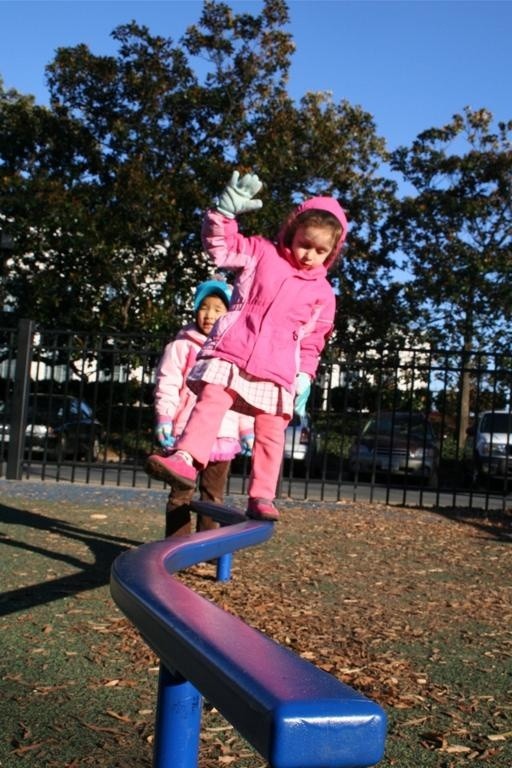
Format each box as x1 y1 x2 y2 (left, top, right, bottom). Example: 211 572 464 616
247 495 283 521
143 452 199 490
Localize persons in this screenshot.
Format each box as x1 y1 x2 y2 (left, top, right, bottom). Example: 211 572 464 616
145 169 350 522
153 279 259 546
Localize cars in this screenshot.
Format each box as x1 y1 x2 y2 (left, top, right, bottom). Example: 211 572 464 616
0 391 107 461
350 410 440 486
474 411 512 475
230 404 320 477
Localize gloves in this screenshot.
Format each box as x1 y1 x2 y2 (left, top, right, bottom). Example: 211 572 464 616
155 417 176 449
216 169 263 217
293 381 311 418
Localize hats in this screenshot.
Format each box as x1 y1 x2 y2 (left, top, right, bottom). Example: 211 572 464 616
191 279 232 316
